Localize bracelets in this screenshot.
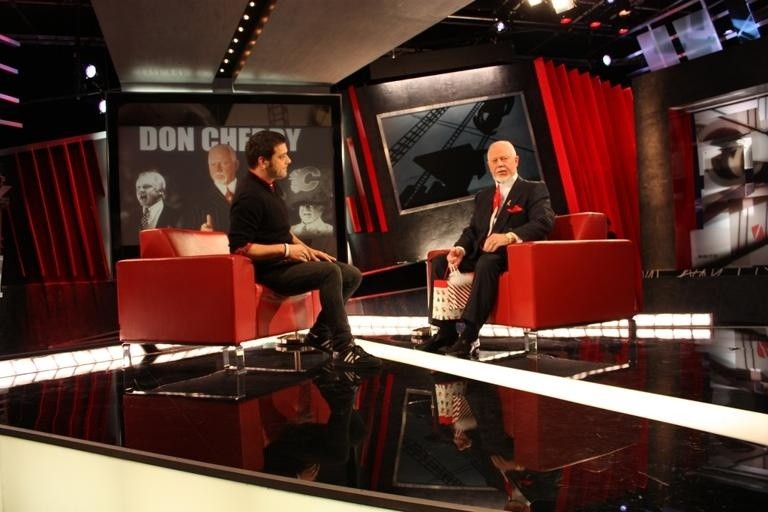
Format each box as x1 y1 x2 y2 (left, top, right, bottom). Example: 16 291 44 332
515 464 521 472
282 242 289 258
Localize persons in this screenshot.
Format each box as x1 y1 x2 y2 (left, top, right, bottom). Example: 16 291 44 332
263 370 364 486
431 364 560 512
228 130 383 370
188 143 242 233
121 170 188 245
413 139 555 359
290 202 334 240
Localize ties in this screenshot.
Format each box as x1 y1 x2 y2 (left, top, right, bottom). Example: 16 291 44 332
141 208 149 230
226 187 234 203
493 184 501 208
504 480 512 500
299 226 312 245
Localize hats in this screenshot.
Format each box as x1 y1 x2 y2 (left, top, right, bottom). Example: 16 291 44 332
289 190 329 207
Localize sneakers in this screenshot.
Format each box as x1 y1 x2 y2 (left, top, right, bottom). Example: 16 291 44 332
304 332 333 353
307 357 363 386
334 345 382 367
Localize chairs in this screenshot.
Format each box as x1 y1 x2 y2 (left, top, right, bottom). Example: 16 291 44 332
426 212 642 353
463 354 643 472
115 229 322 402
123 378 331 474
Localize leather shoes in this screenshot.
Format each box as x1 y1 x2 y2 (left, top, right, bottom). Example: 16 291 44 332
445 334 481 355
415 332 459 351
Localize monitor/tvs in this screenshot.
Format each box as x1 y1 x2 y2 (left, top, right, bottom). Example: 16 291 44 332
392 387 506 492
106 90 347 277
376 91 545 215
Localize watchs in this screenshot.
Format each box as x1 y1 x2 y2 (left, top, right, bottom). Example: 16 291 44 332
505 232 514 244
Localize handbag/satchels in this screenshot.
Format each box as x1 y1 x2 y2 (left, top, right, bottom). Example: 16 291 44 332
435 382 477 431
433 280 472 320
